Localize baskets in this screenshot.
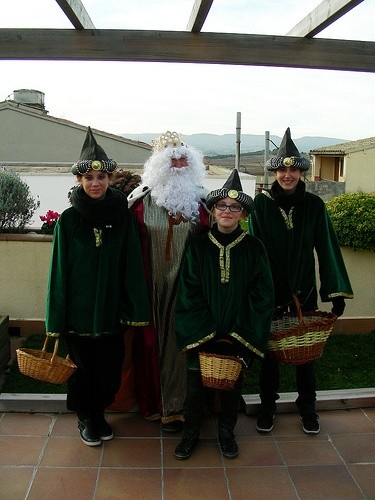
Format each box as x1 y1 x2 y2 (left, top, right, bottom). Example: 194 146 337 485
269 295 338 364
198 339 242 391
16 334 77 385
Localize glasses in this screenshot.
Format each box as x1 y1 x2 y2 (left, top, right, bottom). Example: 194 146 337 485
211 203 243 212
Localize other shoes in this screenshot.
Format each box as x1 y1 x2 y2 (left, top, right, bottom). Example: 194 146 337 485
99 414 114 440
300 410 321 434
162 417 177 433
217 429 239 459
256 410 276 432
77 419 101 446
174 430 201 460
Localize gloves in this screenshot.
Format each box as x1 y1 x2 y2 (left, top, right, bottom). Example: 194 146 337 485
331 296 346 317
202 339 243 355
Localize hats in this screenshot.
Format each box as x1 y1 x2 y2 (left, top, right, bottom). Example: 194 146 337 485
70 125 117 175
206 169 252 216
265 128 310 171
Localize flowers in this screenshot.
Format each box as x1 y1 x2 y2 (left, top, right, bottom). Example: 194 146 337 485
40 209 60 223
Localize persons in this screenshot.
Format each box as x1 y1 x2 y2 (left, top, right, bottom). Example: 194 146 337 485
44 125 156 447
250 127 355 434
105 127 211 434
164 168 281 460
